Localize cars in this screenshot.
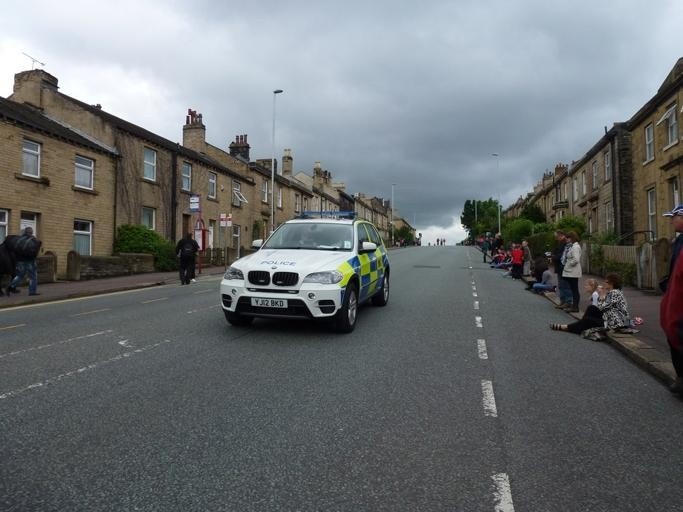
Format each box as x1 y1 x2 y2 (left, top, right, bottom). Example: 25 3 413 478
219 210 391 335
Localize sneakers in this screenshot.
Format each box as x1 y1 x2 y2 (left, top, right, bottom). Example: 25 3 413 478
1 284 42 296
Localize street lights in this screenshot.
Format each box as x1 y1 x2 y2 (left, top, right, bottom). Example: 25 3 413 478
490 150 501 234
269 89 284 233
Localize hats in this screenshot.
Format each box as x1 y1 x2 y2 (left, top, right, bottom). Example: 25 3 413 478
661 206 683 218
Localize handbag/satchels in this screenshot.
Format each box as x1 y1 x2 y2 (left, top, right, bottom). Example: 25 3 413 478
4 234 41 258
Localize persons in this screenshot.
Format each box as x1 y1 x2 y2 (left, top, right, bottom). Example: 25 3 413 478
462 230 629 333
659 246 683 381
6 227 40 297
401 237 445 247
0 269 20 297
175 233 199 285
658 205 683 395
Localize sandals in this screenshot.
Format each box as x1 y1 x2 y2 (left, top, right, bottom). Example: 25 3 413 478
550 322 564 330
